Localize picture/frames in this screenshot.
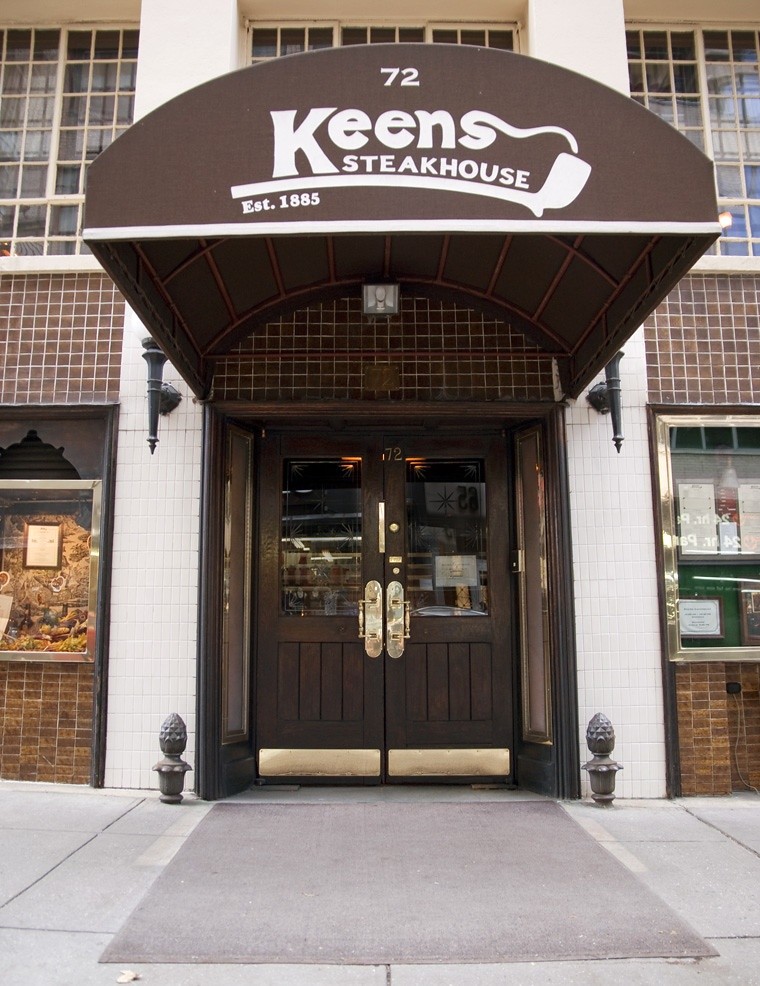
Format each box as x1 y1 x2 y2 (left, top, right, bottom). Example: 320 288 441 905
23 521 63 570
738 578 760 646
679 595 726 640
